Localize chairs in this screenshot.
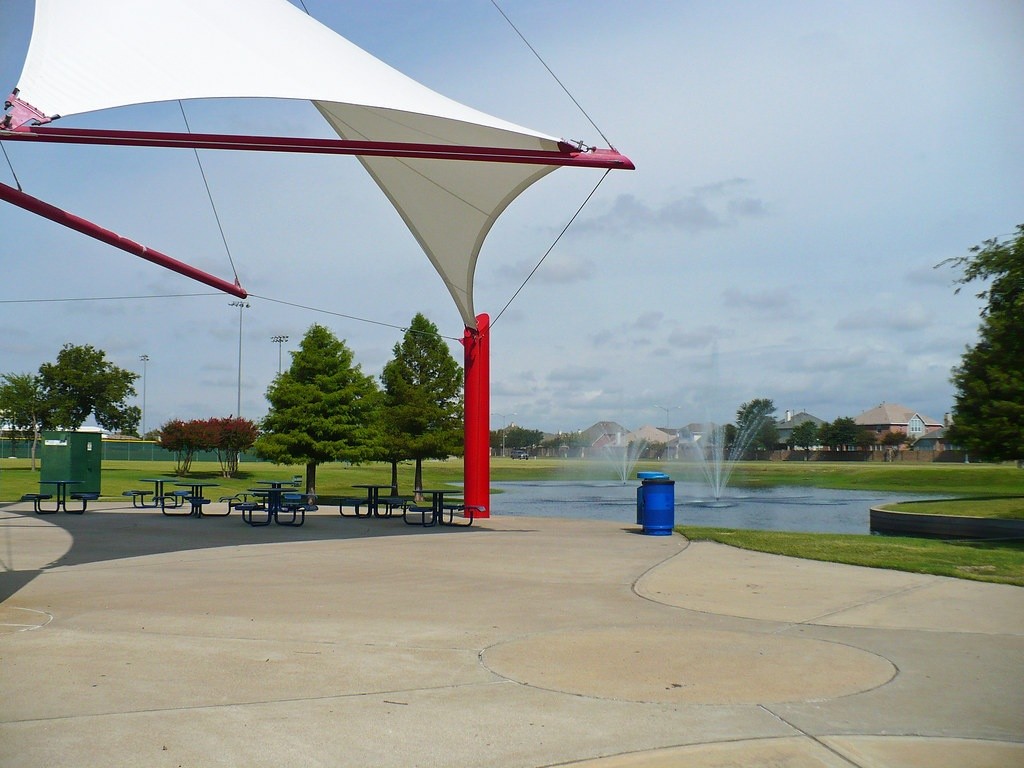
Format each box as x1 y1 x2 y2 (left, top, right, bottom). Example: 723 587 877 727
122 490 145 507
32 493 52 512
240 502 260 525
71 493 99 514
236 504 257 527
174 490 189 506
294 503 319 526
300 493 318 508
444 503 465 526
396 502 417 525
334 497 349 516
236 492 256 511
345 498 369 517
457 505 486 528
154 496 176 516
21 493 43 513
219 496 242 516
133 490 154 508
72 491 102 514
286 501 308 524
409 505 437 528
380 497 404 517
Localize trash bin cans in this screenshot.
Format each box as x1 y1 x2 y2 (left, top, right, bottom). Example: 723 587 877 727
641 478 678 537
635 469 668 525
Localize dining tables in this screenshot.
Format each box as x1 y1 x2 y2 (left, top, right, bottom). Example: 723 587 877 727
37 480 86 513
255 480 296 509
351 484 397 517
413 487 464 525
140 478 181 508
248 486 299 525
175 482 220 515
